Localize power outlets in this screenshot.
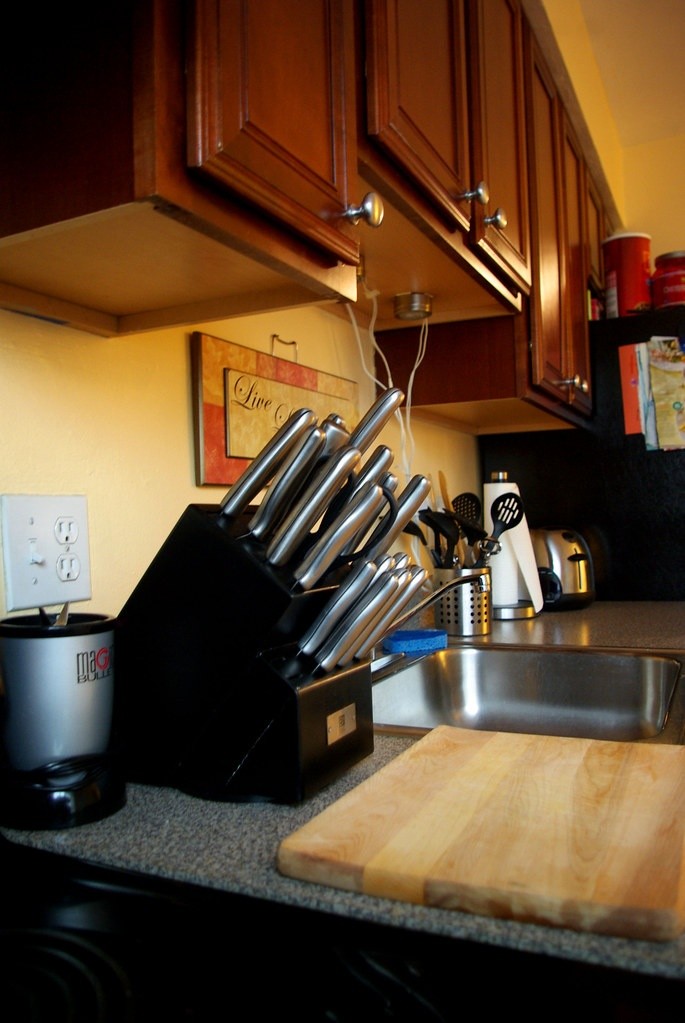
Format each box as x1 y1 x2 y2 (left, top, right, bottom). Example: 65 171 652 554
0 493 91 612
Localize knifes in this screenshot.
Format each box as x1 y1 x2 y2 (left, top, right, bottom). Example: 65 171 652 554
218 387 433 687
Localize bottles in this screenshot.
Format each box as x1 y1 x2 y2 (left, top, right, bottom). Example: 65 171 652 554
651 250 685 312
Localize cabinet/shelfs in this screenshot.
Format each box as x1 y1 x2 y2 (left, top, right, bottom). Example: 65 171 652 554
0 0 632 438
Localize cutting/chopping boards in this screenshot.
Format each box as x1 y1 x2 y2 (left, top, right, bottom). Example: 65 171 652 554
276 724 685 942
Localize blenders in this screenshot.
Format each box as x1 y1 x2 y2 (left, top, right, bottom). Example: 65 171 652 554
0 601 131 834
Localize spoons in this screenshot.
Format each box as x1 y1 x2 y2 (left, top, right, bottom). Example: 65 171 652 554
402 520 438 568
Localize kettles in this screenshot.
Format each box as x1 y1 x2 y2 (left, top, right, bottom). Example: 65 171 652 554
529 528 596 612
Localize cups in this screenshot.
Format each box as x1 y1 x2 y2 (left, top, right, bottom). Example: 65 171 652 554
601 232 653 318
